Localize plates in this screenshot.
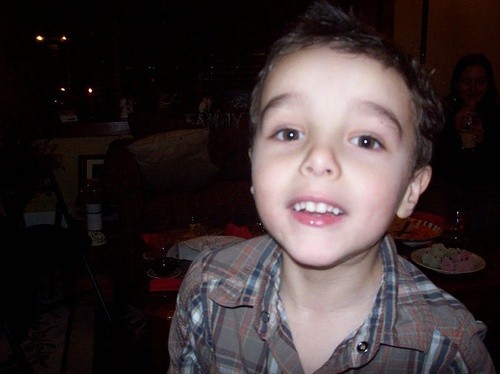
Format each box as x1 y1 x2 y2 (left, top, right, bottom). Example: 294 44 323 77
146 268 182 280
142 252 153 259
387 211 448 241
412 247 486 274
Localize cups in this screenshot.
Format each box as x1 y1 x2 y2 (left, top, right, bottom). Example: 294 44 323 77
151 256 178 276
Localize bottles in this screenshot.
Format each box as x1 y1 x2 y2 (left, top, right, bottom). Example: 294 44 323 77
85 184 104 232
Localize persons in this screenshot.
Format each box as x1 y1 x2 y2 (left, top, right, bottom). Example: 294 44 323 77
166 0 500 373
441 53 498 177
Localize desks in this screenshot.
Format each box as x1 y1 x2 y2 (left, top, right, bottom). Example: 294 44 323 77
111 194 500 348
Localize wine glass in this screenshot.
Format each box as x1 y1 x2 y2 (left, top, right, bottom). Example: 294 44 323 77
461 115 476 150
